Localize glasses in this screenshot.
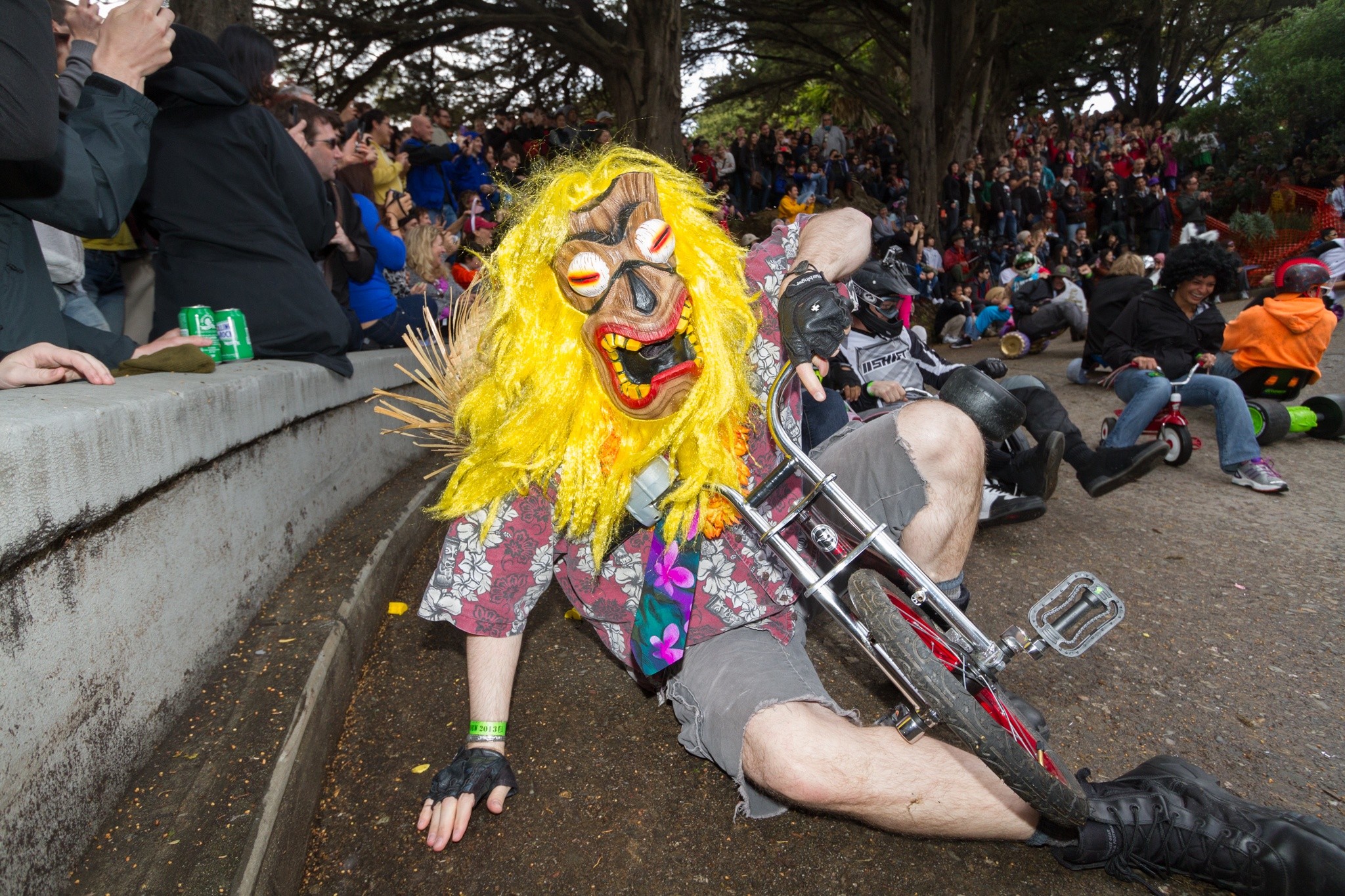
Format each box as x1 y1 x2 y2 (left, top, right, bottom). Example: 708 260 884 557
875 297 904 318
1318 286 1330 295
306 138 335 149
468 200 482 204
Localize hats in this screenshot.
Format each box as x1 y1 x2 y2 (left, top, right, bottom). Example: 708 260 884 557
464 216 496 234
951 234 966 243
1016 231 1030 241
905 215 921 223
785 160 795 168
1148 178 1159 185
463 132 479 140
996 167 1011 176
597 112 612 121
961 215 973 222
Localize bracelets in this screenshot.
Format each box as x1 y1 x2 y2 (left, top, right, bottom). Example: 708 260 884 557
466 734 505 742
867 381 875 396
390 228 399 231
469 720 506 736
1195 353 1202 360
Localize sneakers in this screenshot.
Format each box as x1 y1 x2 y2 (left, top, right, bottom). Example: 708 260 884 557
975 431 1289 527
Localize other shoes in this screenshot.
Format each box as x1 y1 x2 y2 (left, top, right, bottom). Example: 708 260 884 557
932 298 938 305
937 298 943 304
943 334 981 349
827 197 839 208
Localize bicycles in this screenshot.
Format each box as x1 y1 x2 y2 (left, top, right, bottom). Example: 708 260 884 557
604 355 1127 832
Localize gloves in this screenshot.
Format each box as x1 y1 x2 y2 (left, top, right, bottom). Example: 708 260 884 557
975 358 1007 379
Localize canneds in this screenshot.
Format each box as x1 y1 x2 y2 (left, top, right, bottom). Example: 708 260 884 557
214 307 254 363
178 304 222 365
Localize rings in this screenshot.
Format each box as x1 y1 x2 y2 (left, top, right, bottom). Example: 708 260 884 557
1210 362 1214 366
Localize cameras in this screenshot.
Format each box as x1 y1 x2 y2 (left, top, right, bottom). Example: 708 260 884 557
386 188 415 207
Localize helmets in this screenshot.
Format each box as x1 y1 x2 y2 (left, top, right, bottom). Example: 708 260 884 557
1012 252 1036 278
1050 265 1072 279
845 261 921 339
1283 264 1329 293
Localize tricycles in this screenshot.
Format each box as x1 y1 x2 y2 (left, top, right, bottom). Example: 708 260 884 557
999 304 1068 359
1100 362 1206 468
902 371 1030 499
1230 365 1345 446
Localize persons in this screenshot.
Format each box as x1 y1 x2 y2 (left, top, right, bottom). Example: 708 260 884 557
0 0 1345 527
369 137 1345 896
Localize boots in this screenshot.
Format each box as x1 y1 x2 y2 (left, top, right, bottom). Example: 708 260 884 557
1054 754 1344 896
902 585 1051 743
